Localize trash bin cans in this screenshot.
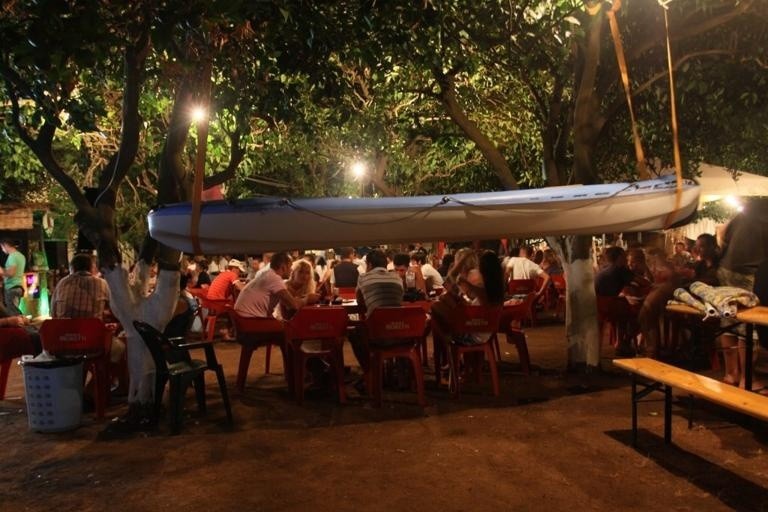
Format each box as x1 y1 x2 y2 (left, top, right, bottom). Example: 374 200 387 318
20 357 83 433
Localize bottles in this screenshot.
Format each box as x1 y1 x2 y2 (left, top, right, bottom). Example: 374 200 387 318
406 266 416 289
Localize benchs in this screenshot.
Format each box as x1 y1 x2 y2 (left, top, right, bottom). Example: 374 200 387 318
614 355 768 442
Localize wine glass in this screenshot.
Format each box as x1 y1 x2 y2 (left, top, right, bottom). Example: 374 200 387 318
325 283 334 306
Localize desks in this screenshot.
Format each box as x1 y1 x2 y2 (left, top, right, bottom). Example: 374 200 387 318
664 301 768 421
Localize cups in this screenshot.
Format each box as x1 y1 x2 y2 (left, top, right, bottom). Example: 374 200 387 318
333 287 339 295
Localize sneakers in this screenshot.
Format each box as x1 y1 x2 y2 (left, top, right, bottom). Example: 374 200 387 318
110 386 128 402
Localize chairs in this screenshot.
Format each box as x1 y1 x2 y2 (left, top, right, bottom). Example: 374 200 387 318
594 265 708 371
1 316 128 427
188 287 235 341
508 275 564 323
223 287 536 408
132 319 235 434
